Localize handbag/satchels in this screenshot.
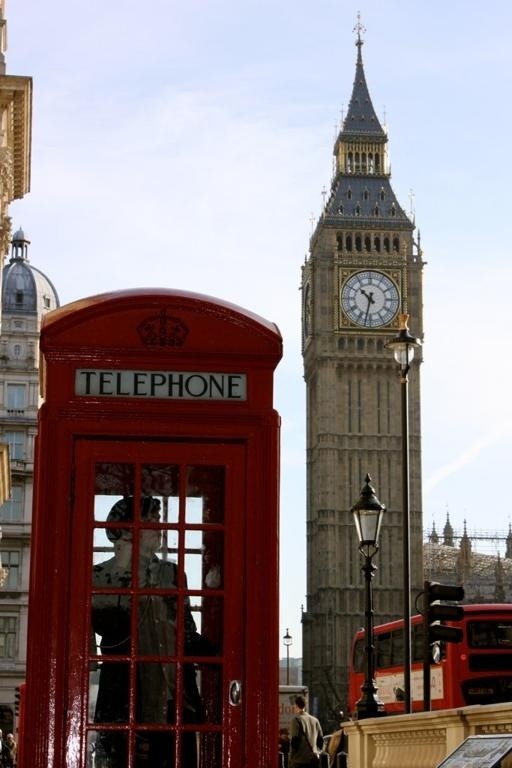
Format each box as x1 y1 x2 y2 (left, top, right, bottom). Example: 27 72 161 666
320 751 329 768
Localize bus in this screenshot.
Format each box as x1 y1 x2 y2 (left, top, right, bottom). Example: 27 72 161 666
348 603 512 724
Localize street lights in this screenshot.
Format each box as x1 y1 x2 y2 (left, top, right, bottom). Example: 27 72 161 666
388 307 422 715
350 473 388 723
283 629 292 683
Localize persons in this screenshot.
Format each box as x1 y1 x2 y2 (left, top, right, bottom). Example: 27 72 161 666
92 490 217 767
287 693 323 768
278 729 290 767
0 729 10 768
4 733 16 764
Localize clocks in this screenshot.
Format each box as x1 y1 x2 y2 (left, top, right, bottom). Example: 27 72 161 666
340 269 402 329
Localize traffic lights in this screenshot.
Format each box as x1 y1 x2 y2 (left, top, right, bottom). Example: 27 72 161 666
423 579 467 666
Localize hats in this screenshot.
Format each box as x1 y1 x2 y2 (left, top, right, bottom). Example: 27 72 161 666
105 497 160 542
279 728 290 736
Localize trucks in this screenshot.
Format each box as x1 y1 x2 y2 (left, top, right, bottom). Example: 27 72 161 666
273 685 312 754
